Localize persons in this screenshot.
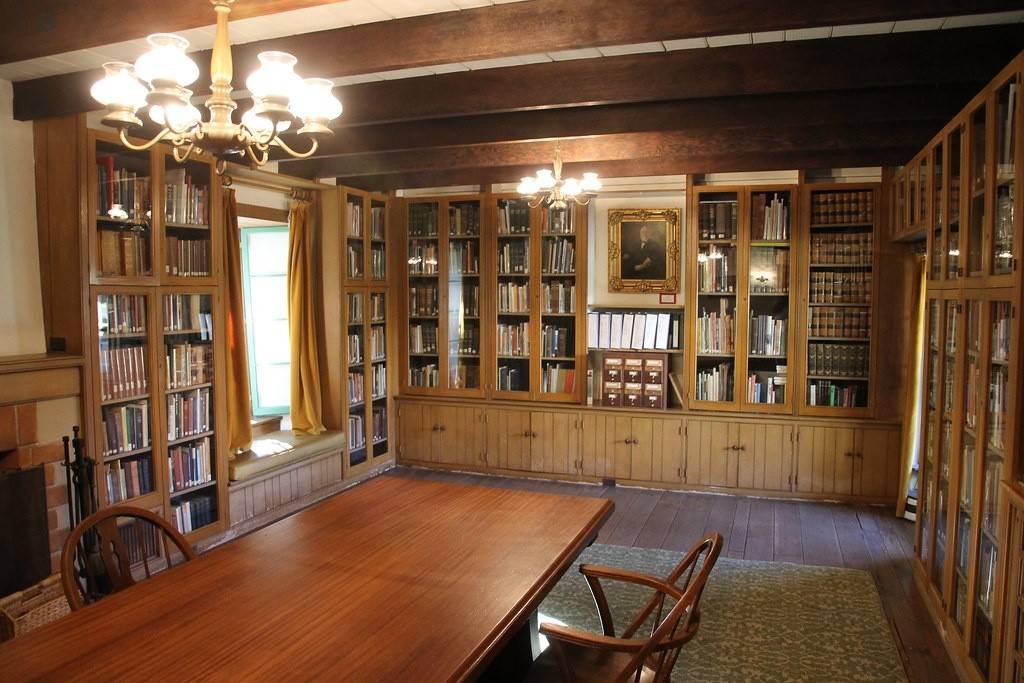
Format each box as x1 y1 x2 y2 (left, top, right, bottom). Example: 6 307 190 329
621 225 665 280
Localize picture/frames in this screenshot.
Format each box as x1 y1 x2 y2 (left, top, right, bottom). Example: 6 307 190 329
608 205 682 294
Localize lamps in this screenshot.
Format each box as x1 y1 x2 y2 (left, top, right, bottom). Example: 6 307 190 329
87 0 341 175
517 141 603 211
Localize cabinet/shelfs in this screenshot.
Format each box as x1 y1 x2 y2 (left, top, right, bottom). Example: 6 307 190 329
36 46 1023 683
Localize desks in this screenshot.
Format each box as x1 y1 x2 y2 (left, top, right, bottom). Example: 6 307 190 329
0 474 616 683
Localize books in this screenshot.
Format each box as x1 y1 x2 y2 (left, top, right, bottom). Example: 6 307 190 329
696 191 873 407
667 371 683 408
97 153 218 534
587 367 594 405
587 312 681 350
916 183 1024 683
346 199 577 451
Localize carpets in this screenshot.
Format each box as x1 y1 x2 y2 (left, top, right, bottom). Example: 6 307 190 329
536 544 910 683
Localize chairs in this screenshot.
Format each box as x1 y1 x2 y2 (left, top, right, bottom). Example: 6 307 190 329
517 530 724 683
60 504 197 614
